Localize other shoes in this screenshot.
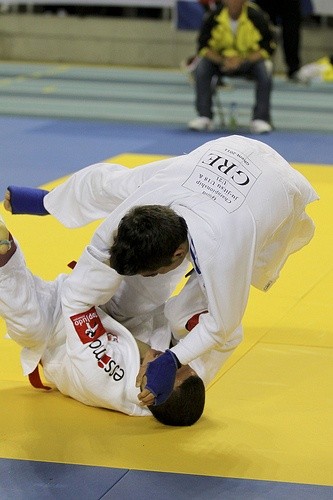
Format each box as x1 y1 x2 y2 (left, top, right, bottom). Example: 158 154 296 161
250 119 273 135
188 116 214 131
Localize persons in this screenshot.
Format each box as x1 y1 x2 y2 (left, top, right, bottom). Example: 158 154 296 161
185 0 307 134
3 135 319 408
0 222 243 427
